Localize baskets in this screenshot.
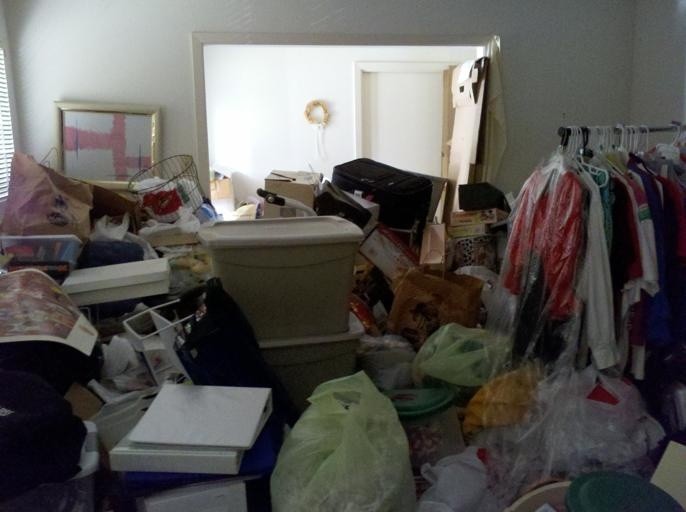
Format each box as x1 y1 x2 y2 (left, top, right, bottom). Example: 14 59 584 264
128 154 204 224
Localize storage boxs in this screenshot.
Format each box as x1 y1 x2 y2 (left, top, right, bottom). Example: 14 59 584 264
258 312 368 415
56 257 172 307
263 170 323 218
199 218 365 339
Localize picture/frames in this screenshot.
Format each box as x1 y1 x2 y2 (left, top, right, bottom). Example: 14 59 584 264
55 100 161 192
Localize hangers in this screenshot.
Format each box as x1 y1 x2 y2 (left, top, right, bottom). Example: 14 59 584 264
544 118 686 188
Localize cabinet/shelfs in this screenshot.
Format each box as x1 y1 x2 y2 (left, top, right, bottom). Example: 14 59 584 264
209 173 235 214
